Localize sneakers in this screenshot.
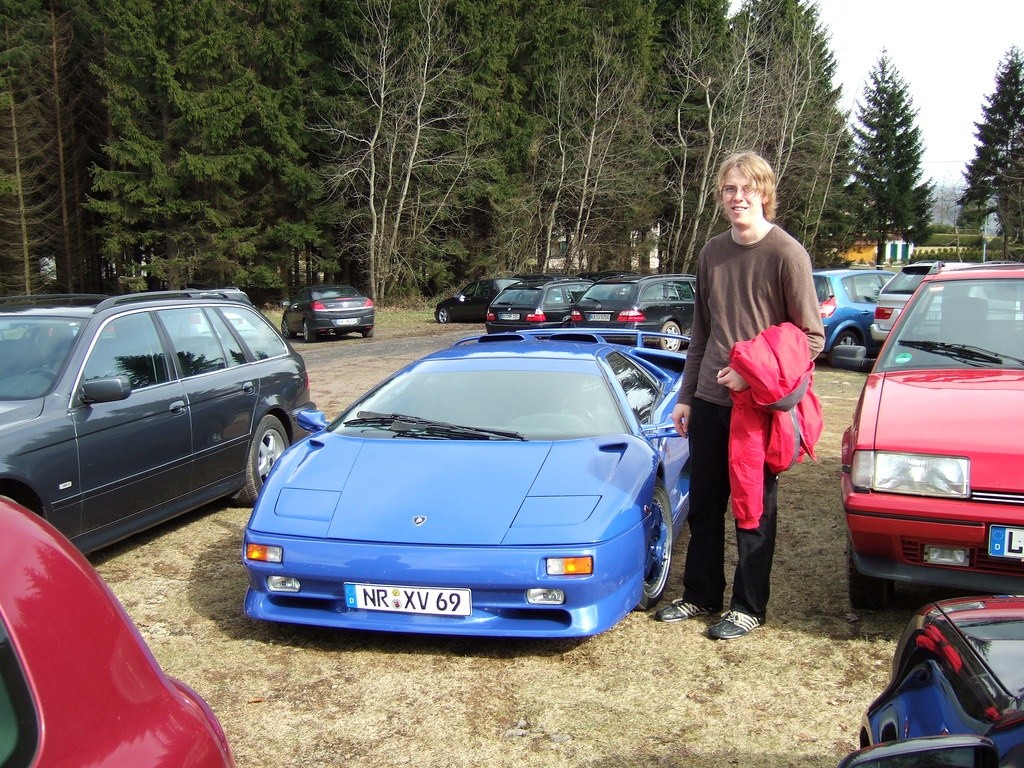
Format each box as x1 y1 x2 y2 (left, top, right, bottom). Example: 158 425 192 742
656 598 722 623
709 610 768 642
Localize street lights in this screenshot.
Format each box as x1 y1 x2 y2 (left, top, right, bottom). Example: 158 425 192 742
512 269 637 282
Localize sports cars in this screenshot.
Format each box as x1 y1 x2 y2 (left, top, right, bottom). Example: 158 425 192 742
243 321 690 640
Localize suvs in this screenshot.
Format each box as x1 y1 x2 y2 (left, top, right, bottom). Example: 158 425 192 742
869 261 1024 343
837 260 1024 613
483 277 597 334
569 274 697 353
810 270 910 356
0 286 317 556
434 277 526 323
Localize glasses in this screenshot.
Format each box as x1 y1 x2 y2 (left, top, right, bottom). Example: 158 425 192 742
721 186 761 194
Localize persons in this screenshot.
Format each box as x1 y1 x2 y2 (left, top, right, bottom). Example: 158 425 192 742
652 152 827 641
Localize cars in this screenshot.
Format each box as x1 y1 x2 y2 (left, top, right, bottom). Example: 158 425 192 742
834 591 1024 768
280 285 376 343
0 495 236 768
